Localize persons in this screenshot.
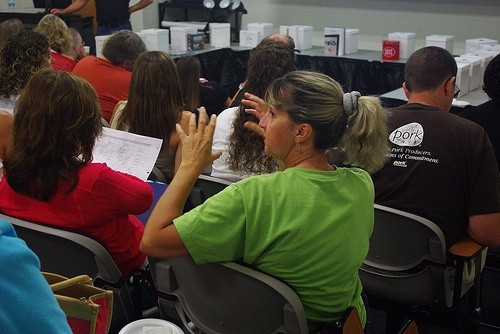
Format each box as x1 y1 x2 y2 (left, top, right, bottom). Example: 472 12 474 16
0 218 74 334
50 0 153 36
140 71 392 334
0 13 213 184
1 67 156 286
367 45 500 308
208 32 297 184
462 52 500 173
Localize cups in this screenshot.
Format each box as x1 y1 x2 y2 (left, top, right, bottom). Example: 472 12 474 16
119 318 185 334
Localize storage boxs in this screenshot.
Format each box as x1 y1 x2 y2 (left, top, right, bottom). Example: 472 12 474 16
95 34 114 61
170 26 198 52
381 31 416 62
240 22 274 52
426 34 455 57
454 37 500 98
209 22 231 48
324 26 360 56
279 24 313 50
142 28 169 54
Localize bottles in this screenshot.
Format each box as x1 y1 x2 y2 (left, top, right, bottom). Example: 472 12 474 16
7 0 17 12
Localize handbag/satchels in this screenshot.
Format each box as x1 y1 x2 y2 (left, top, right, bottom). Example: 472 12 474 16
42 272 113 334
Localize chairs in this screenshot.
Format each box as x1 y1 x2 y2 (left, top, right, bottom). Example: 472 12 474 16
0 116 500 334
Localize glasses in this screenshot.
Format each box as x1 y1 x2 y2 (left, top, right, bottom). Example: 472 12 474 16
454 82 459 99
81 41 85 46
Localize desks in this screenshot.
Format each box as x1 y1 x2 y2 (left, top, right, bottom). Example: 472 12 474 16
169 40 494 118
0 10 96 56
